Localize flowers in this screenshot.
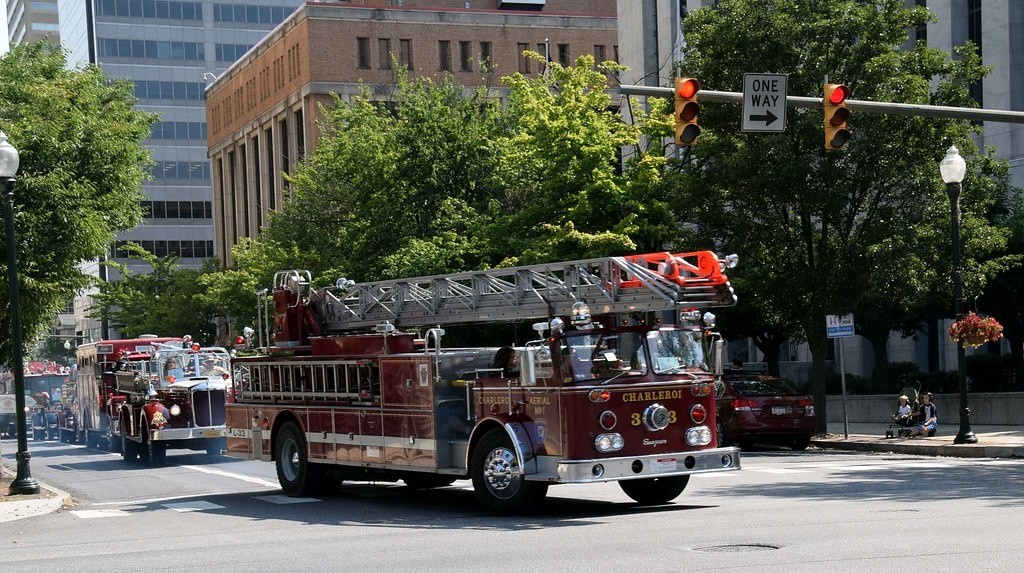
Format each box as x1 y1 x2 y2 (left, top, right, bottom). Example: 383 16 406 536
947 310 1004 350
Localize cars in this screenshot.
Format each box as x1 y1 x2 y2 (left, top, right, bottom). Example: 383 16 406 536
716 371 816 451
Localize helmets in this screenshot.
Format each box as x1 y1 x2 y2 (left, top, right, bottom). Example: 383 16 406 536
923 392 932 396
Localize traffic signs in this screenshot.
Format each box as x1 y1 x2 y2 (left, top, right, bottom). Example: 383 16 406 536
741 74 788 133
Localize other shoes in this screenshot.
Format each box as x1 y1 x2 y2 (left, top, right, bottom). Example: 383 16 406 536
893 417 898 420
928 428 937 437
908 434 913 439
901 415 907 419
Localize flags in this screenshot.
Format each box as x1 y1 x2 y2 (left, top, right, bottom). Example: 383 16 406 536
542 51 559 91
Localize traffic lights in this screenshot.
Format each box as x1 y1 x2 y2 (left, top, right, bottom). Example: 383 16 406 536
674 77 701 145
824 83 850 150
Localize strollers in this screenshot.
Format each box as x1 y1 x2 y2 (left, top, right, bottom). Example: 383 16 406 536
886 380 921 438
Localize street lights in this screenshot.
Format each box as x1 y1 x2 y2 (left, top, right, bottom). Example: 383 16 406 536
0 130 39 494
940 144 978 444
63 339 70 363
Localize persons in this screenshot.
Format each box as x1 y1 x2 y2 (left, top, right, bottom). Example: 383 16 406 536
163 359 185 380
561 348 582 377
677 332 703 366
894 395 912 424
201 356 228 375
909 392 938 437
55 389 62 398
491 347 520 378
732 358 743 369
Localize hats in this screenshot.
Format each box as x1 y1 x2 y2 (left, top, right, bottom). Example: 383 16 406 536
201 356 218 366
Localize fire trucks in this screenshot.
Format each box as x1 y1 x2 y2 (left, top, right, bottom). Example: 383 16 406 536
219 251 743 516
0 334 231 469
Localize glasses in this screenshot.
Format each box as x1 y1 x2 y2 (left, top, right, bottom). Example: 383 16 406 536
169 362 175 364
